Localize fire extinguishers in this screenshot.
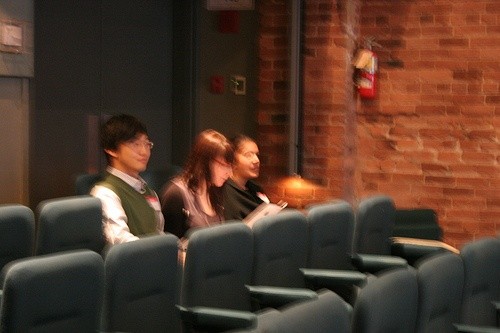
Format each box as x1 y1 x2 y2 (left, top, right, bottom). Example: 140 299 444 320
359 38 382 99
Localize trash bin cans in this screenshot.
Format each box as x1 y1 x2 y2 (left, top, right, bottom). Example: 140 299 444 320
270 175 330 209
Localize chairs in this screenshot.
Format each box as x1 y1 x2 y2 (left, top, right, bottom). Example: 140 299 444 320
0 146 500 333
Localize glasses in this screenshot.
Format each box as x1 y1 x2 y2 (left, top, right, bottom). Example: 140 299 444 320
121 141 152 150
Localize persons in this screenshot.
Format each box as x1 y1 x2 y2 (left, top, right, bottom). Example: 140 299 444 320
219 134 271 225
89 114 186 270
160 128 235 247
